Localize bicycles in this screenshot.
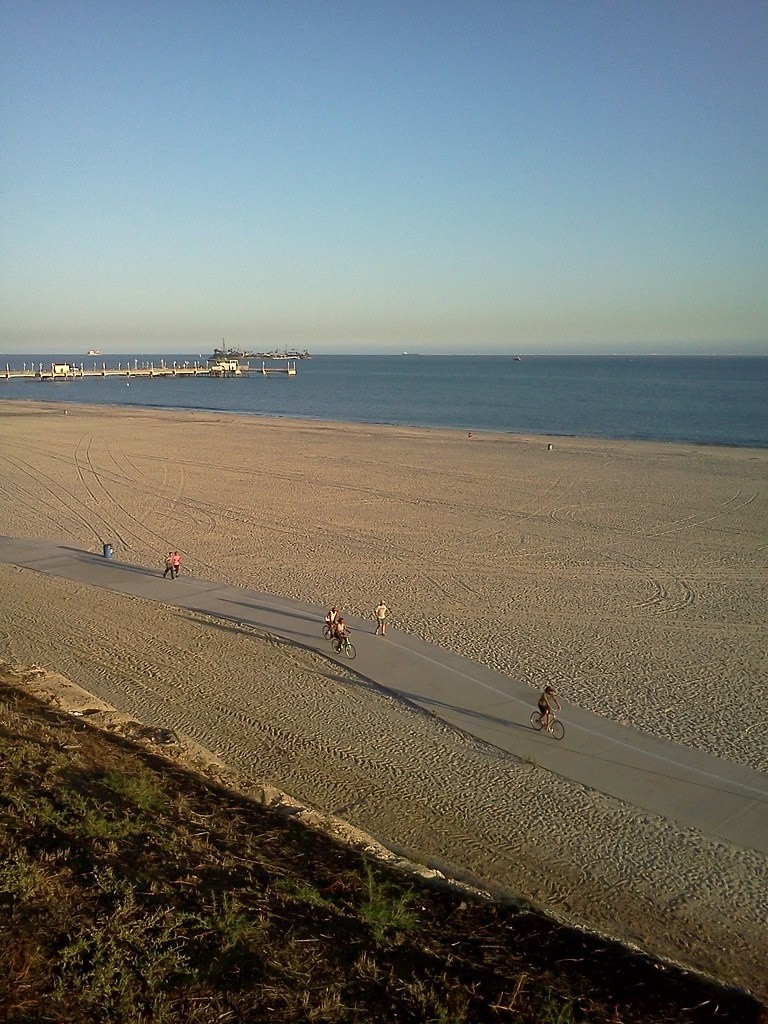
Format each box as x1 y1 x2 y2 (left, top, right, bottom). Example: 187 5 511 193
332 634 356 660
530 709 565 740
322 620 337 639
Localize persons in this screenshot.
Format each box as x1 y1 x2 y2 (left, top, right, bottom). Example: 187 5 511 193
334 618 351 652
538 686 561 731
374 600 389 636
172 551 181 577
325 608 340 637
163 552 175 579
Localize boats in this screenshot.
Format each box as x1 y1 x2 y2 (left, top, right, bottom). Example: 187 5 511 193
513 357 521 360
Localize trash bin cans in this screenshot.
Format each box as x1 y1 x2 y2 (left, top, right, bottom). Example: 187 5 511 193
104 543 113 559
